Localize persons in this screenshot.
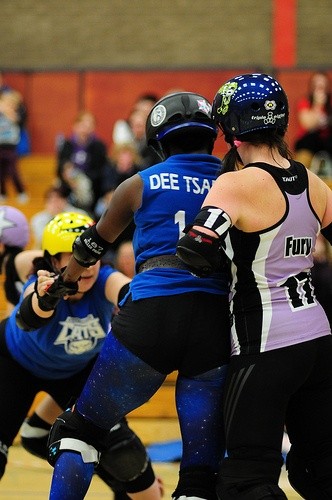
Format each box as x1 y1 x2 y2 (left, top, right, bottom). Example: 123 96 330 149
0 205 43 323
0 211 164 500
292 69 332 172
0 69 30 204
49 91 285 499
53 94 159 219
182 72 331 500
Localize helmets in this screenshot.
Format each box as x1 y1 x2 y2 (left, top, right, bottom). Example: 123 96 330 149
41 211 97 256
146 92 219 146
0 206 29 248
212 73 289 135
